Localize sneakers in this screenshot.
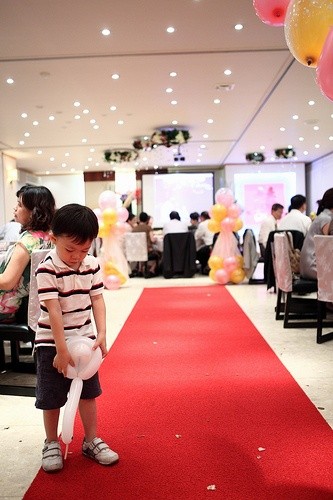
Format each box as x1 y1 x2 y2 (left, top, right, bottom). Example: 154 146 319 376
81 436 119 465
42 437 63 473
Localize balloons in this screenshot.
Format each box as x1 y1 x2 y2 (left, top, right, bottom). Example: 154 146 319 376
253 0 291 27
208 257 245 284
317 28 333 101
102 263 126 290
61 336 102 444
284 0 333 68
208 188 243 233
93 191 128 239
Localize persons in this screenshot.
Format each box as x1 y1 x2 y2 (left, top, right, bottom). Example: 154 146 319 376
163 210 188 233
299 188 333 281
195 211 214 273
134 212 160 272
277 194 312 237
0 184 55 325
33 203 118 471
259 203 284 248
189 213 200 229
125 214 135 232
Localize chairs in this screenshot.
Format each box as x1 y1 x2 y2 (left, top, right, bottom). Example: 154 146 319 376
0 248 59 403
124 230 333 345
